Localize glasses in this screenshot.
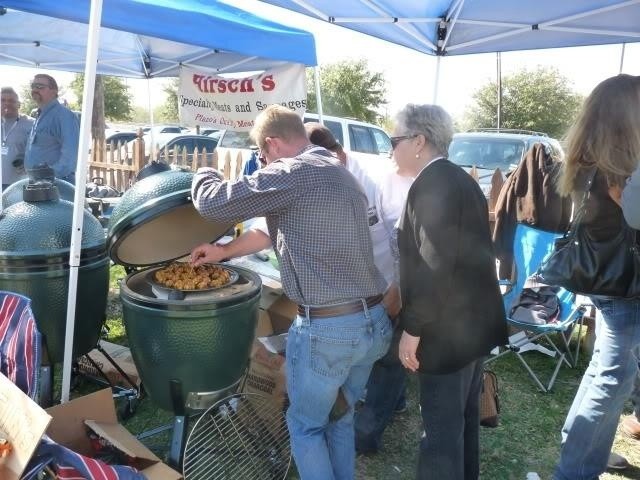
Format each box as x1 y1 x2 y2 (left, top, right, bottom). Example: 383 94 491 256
258 141 268 164
31 82 48 90
389 134 417 149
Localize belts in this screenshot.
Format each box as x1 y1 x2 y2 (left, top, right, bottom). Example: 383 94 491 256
292 293 384 317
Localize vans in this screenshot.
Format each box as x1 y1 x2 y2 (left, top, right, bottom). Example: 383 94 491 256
212 112 394 189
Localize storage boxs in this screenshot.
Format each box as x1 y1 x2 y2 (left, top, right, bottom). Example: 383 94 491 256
1 338 183 480
237 284 297 442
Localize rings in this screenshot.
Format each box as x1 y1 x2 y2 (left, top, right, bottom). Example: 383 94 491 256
405 357 409 360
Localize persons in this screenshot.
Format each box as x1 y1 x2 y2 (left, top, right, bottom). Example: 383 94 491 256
2 86 36 191
24 73 80 202
190 121 407 454
552 72 640 480
189 102 394 480
390 102 512 480
608 157 640 439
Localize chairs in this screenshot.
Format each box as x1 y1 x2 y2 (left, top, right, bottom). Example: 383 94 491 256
483 223 587 393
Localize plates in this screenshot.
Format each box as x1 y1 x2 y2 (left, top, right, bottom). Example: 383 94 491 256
144 262 240 291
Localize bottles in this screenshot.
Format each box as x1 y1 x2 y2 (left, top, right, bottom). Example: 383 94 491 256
12 159 25 178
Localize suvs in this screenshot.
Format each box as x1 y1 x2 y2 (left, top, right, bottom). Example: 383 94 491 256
446 127 566 203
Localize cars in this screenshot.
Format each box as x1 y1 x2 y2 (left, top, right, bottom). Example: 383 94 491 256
30 108 223 192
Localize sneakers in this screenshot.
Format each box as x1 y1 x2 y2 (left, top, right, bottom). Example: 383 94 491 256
620 415 640 438
606 452 628 469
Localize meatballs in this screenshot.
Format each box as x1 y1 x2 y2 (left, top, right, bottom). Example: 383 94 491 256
155 264 229 290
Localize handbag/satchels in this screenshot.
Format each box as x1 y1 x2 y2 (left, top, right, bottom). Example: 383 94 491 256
540 165 639 301
476 369 499 428
